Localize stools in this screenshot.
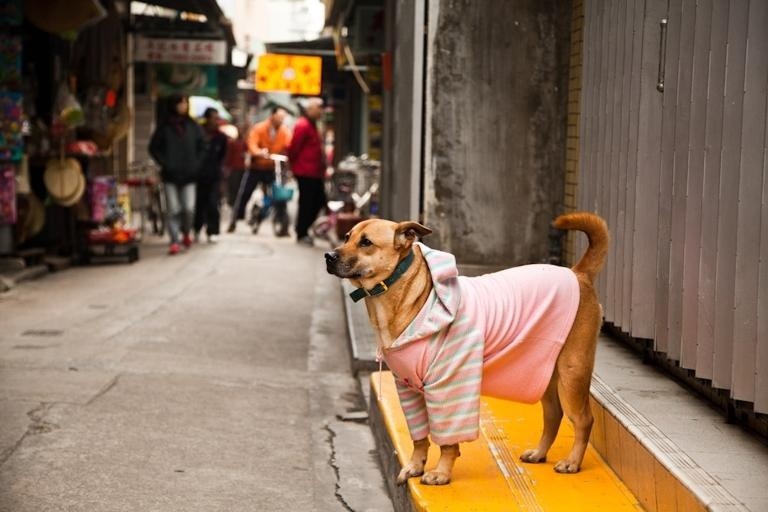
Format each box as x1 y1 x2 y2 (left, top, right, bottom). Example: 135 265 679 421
122 175 163 236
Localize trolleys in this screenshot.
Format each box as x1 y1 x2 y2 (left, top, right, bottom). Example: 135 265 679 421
71 167 145 264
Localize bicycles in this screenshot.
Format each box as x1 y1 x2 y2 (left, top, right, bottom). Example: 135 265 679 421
250 154 294 236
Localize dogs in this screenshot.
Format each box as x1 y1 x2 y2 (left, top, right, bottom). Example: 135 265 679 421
321 209 612 487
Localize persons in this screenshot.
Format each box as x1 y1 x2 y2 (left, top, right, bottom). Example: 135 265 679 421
148 92 327 256
